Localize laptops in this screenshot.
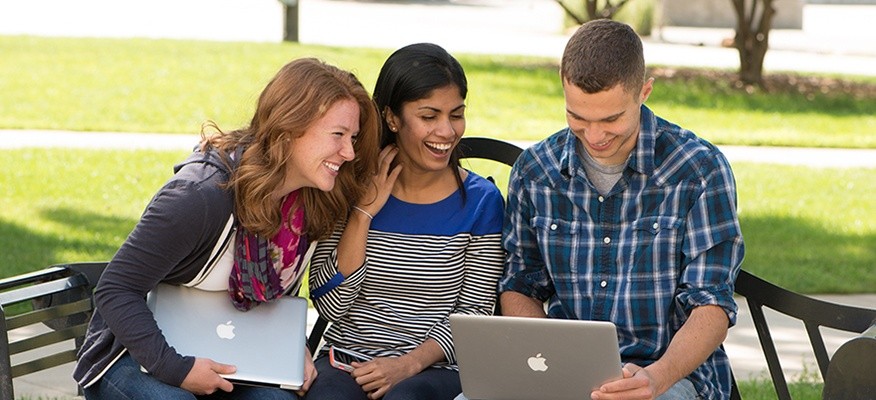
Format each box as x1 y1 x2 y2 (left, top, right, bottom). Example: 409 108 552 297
450 313 623 400
139 283 309 391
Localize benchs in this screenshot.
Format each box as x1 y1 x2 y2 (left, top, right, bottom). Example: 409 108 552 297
0 137 876 400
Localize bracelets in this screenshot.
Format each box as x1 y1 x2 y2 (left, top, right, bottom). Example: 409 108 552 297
353 205 373 219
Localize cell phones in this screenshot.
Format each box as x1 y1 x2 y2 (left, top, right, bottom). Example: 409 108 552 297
329 346 373 372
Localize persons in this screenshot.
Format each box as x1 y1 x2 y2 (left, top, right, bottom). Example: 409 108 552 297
453 19 745 400
306 42 505 400
74 58 379 400
